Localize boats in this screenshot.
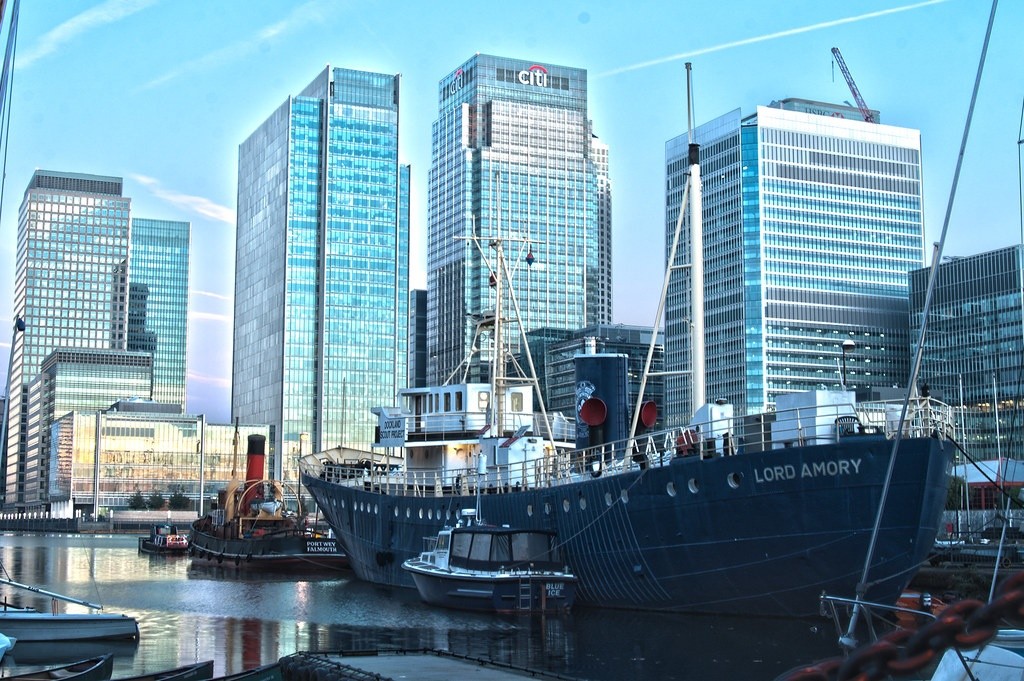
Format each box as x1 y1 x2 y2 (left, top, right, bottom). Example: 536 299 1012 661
400 460 578 620
115 659 215 680
138 523 188 555
1 561 140 645
185 475 348 573
293 60 959 618
202 660 288 681
0 651 113 681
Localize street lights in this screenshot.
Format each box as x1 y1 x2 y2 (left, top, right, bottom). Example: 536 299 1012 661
298 432 310 518
198 413 208 518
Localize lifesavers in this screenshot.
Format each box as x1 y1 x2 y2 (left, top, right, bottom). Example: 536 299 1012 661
1000 557 1012 568
588 451 602 478
